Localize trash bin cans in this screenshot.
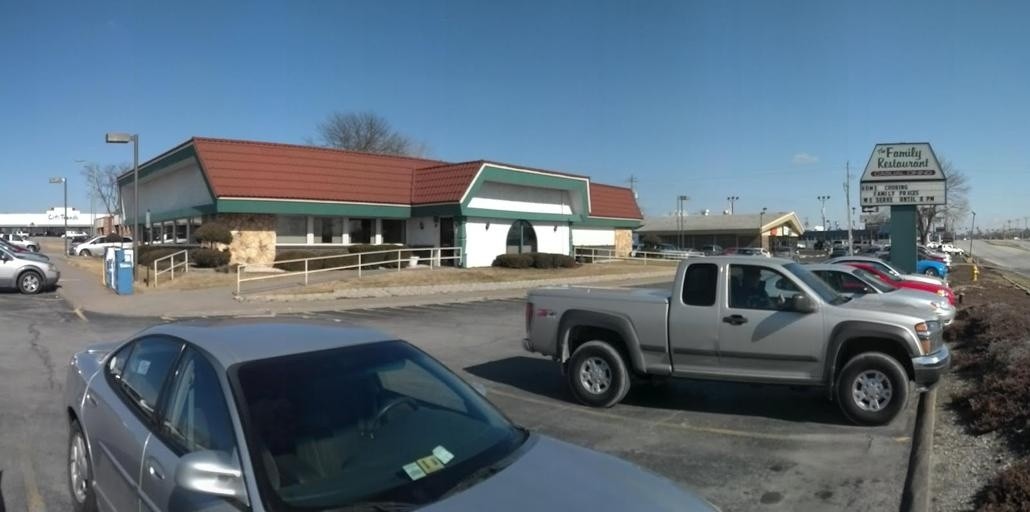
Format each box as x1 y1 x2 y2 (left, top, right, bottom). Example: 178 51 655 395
103 247 134 295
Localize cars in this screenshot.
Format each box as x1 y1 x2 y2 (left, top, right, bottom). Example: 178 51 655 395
0 231 61 296
70 233 135 259
60 317 719 512
807 247 960 328
64 230 87 243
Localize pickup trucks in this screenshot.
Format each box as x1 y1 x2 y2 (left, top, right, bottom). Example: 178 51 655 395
521 253 953 421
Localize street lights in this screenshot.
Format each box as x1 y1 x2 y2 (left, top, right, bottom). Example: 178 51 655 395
48 176 69 258
725 196 738 211
107 132 142 283
817 195 830 244
